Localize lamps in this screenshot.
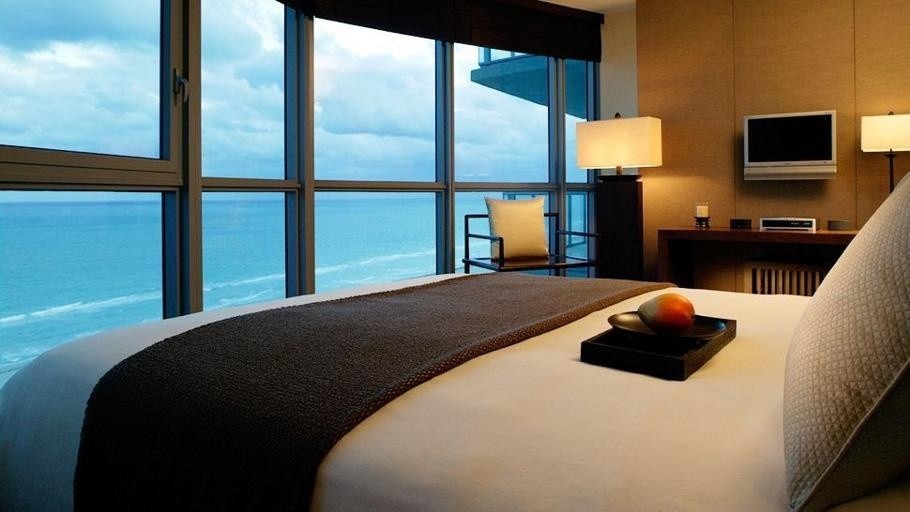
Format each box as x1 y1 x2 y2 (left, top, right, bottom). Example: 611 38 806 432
860 110 910 193
575 113 662 175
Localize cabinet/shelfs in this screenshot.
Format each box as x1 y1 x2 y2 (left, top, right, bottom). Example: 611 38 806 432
593 183 644 281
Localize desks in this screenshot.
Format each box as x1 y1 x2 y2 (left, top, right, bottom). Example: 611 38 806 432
658 226 859 283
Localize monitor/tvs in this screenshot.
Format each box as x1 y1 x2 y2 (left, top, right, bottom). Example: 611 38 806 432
744 110 837 166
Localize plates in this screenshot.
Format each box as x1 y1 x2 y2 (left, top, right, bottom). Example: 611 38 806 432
606 311 728 340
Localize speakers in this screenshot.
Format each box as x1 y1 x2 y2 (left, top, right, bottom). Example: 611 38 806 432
828 220 853 230
730 219 751 228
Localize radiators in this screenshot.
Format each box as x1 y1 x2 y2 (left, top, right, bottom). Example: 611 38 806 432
744 265 819 296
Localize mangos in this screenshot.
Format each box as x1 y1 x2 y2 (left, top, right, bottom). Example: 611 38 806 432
636 293 697 336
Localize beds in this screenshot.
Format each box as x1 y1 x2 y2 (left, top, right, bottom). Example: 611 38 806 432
0 174 910 512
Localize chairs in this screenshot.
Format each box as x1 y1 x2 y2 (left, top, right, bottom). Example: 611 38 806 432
464 209 595 276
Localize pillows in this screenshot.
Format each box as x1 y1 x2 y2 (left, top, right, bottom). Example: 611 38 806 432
484 195 550 260
781 173 910 512
